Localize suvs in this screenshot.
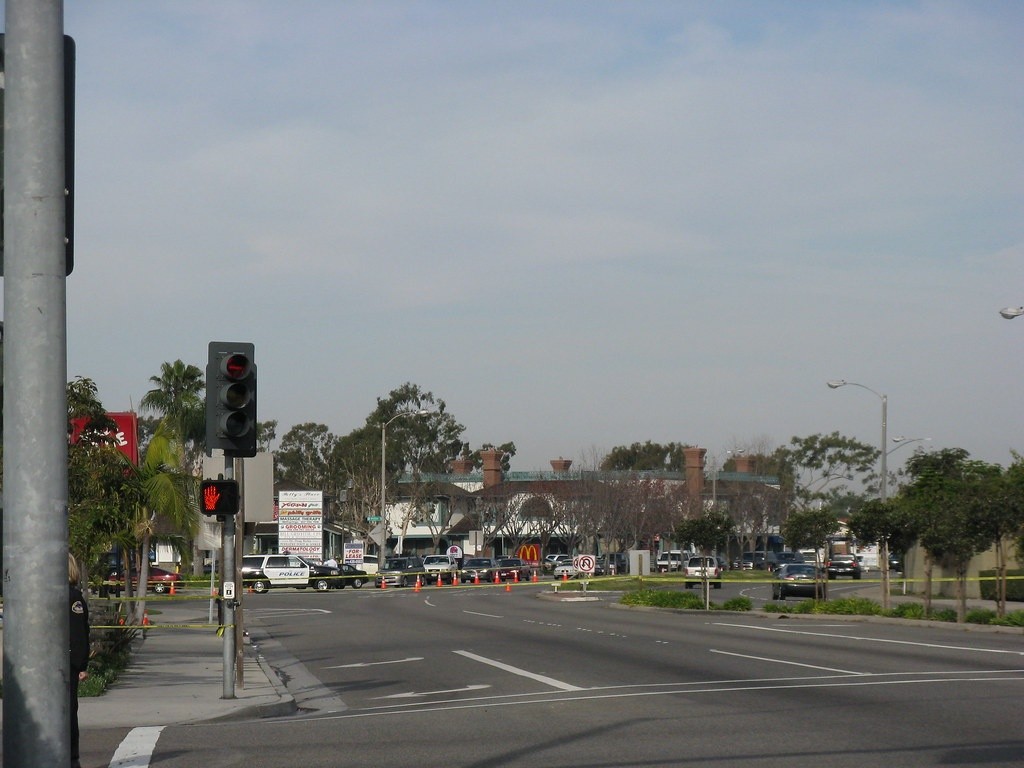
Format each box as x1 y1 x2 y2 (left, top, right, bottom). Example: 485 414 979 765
684 555 721 589
716 551 805 572
656 550 690 572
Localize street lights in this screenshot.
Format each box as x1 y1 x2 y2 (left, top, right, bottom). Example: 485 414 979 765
713 449 746 505
826 380 934 506
378 410 430 572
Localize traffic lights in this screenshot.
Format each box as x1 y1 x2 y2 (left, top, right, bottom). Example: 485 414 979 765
206 342 254 448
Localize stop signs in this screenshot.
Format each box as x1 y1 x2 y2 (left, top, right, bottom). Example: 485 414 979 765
199 480 240 515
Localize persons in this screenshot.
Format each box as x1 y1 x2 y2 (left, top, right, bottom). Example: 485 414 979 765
68 551 89 768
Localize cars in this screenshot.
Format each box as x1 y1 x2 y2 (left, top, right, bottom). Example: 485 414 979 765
109 567 185 594
192 555 389 594
375 555 532 588
539 553 630 579
827 553 862 580
771 564 827 600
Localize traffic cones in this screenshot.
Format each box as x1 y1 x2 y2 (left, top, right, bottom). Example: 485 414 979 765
170 582 175 594
249 586 253 593
143 610 148 625
513 569 567 583
381 570 511 593
213 588 217 595
